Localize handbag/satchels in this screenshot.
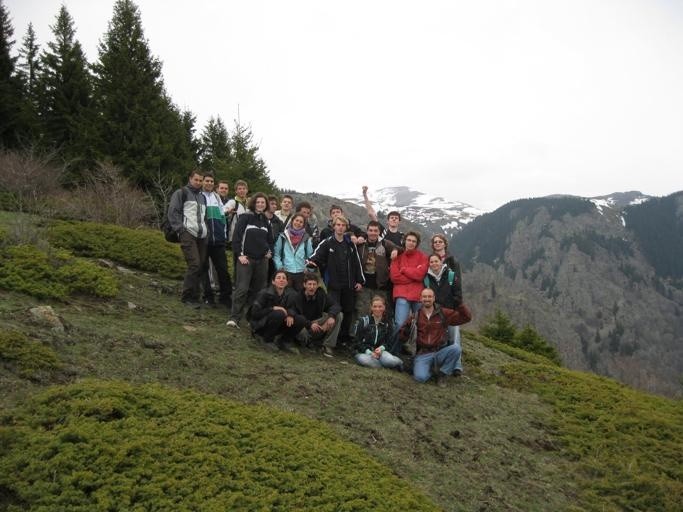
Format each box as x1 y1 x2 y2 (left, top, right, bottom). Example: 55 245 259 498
403 319 417 358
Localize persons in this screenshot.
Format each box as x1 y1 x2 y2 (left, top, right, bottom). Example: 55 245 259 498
389 230 429 329
345 220 404 340
349 295 404 372
430 234 462 284
362 185 405 247
399 287 472 389
422 253 463 378
167 169 369 359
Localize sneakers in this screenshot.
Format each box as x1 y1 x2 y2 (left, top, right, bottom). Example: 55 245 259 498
226 321 241 330
437 376 447 387
454 369 461 377
187 296 233 309
266 342 333 358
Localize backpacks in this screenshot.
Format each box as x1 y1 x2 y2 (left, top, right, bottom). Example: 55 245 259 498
160 187 206 243
